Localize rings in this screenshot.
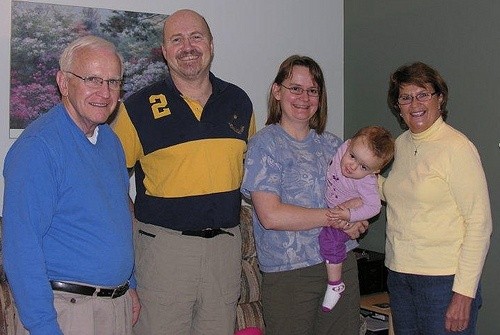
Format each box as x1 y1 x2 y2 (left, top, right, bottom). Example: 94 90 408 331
346 222 349 227
457 325 465 330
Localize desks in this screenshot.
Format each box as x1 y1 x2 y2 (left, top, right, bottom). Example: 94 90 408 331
360 291 395 335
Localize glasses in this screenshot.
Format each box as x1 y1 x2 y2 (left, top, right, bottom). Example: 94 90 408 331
64 71 124 90
396 90 439 105
280 83 321 97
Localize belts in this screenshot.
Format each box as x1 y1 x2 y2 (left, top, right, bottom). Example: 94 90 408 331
182 228 234 239
49 281 130 299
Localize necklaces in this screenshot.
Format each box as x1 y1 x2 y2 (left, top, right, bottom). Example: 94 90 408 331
410 134 433 155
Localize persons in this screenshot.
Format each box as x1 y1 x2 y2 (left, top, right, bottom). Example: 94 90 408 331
318 125 395 312
2 35 141 335
241 54 369 335
377 61 492 335
110 9 255 335
